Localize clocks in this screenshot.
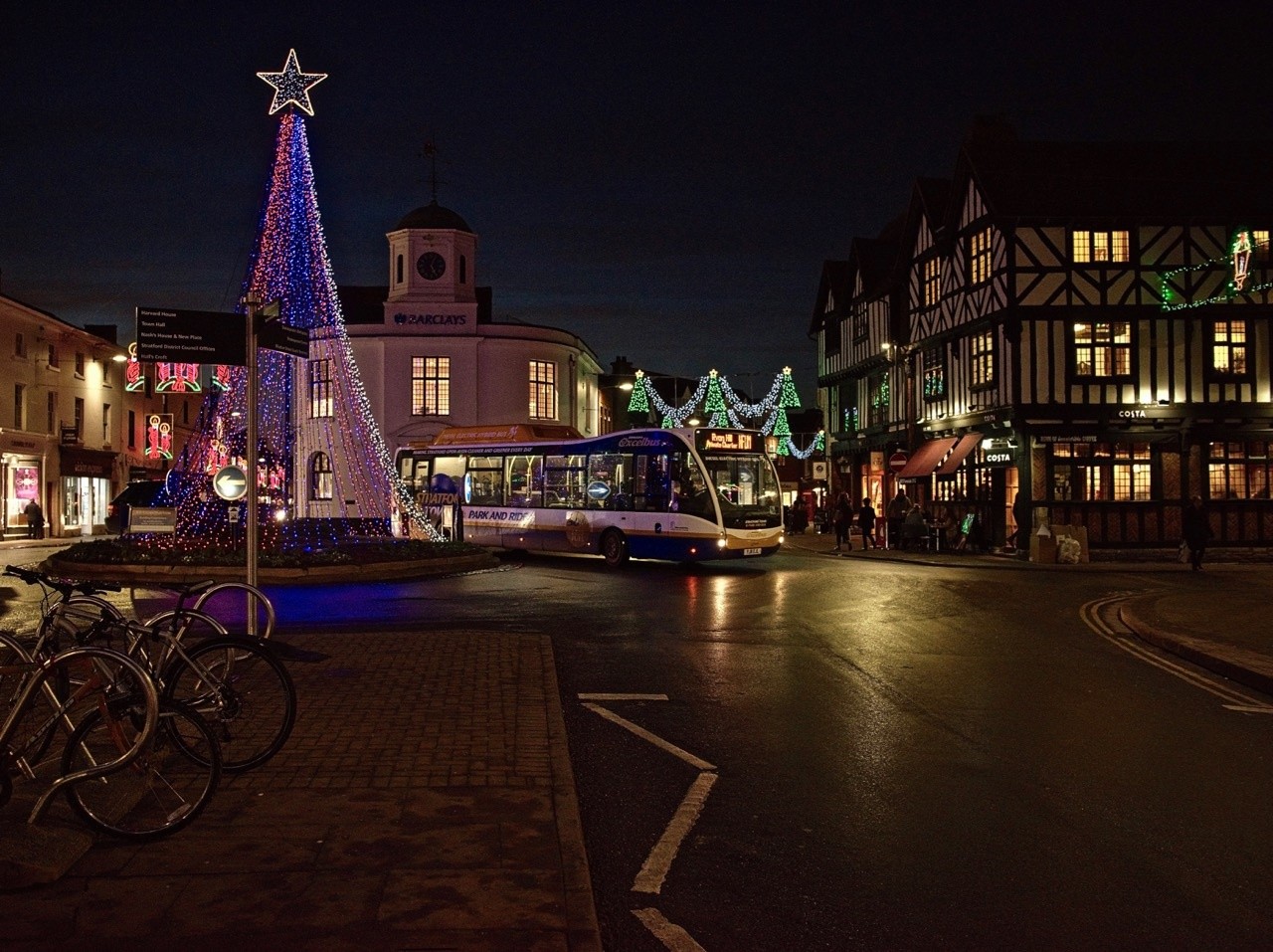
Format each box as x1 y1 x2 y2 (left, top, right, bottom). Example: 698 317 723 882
416 251 446 281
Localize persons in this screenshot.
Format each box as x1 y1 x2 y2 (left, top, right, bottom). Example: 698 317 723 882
858 498 878 550
832 493 854 551
887 489 923 549
788 497 808 538
24 498 40 540
73 486 96 525
1182 495 1212 574
1007 486 1020 547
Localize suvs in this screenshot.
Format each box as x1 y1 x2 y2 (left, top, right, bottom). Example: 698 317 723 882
104 479 212 534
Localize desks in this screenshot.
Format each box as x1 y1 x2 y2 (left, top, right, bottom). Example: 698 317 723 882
930 524 948 551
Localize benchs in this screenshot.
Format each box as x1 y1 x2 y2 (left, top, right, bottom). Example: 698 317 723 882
902 523 932 554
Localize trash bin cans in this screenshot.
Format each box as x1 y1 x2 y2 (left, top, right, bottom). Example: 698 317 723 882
849 528 863 553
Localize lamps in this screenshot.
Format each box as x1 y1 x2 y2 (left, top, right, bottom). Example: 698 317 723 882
882 343 903 349
93 355 127 362
598 378 634 391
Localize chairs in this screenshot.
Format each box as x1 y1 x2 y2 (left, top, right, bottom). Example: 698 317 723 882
889 517 906 548
403 476 636 511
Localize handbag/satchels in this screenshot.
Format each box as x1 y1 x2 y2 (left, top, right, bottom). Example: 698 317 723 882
832 509 844 526
1169 540 1190 564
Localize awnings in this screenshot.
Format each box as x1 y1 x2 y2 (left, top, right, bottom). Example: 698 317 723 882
895 431 984 478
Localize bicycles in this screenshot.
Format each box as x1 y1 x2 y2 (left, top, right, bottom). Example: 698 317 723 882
0 566 331 844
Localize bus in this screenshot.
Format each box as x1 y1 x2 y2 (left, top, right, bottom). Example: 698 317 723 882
390 428 785 568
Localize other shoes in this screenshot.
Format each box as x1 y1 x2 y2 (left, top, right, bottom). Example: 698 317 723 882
1008 538 1014 546
834 546 841 551
1194 566 1204 572
862 547 867 550
872 541 877 549
848 543 852 551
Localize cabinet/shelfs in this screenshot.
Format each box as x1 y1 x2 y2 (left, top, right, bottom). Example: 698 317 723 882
1030 525 1089 563
